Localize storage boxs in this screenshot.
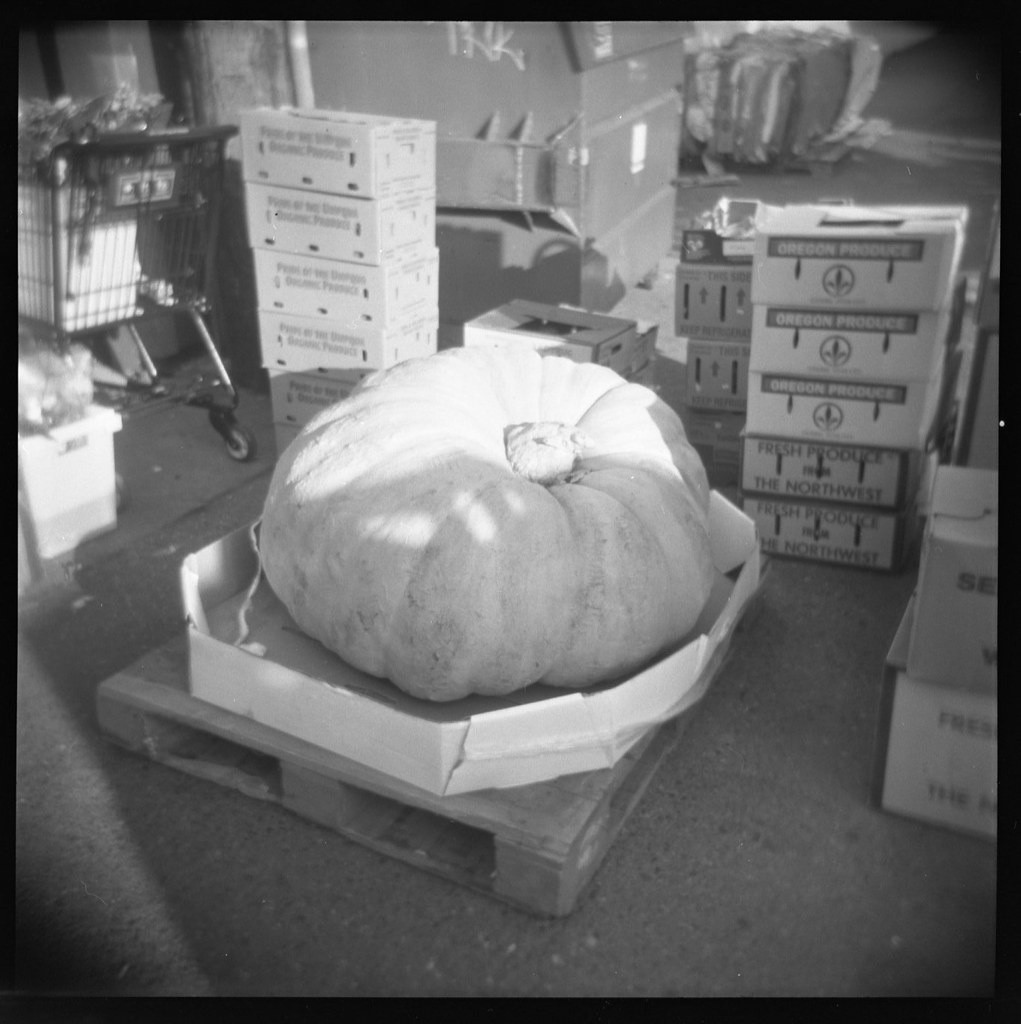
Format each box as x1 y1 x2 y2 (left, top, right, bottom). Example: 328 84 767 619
462 300 658 389
239 104 441 457
742 205 982 570
17 404 125 559
17 185 142 330
964 223 1002 466
678 197 766 411
884 464 998 837
179 489 763 797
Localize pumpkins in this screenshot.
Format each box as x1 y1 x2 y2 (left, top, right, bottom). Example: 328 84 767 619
258 343 716 700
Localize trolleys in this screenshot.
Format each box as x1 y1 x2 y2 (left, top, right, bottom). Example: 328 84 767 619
19 121 258 464
